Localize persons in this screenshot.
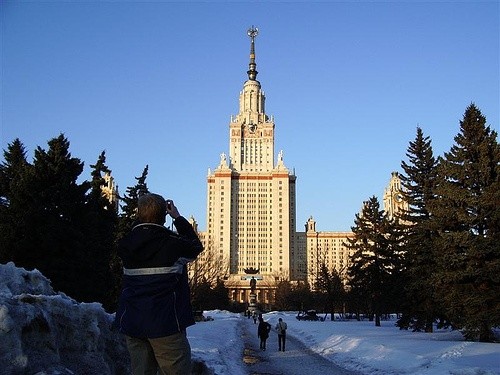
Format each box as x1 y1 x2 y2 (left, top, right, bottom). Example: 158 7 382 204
253 313 258 324
251 310 255 319
117 192 204 374
250 276 256 295
258 318 271 351
247 309 251 319
274 318 287 352
258 310 262 318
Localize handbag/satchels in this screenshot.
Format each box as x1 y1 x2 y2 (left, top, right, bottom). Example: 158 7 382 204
281 330 286 334
